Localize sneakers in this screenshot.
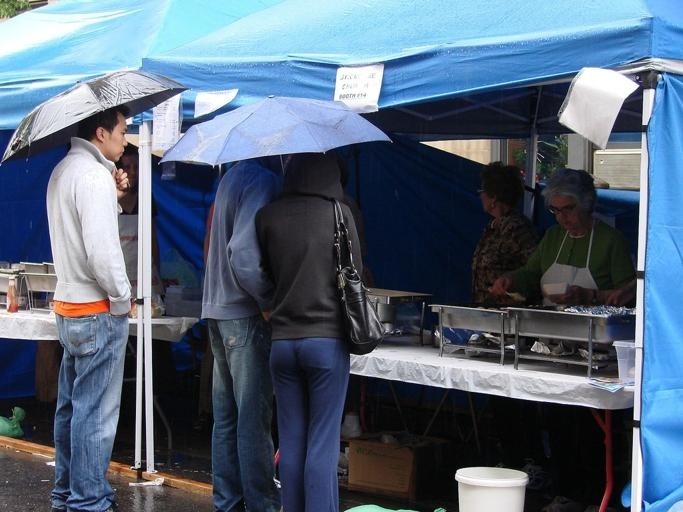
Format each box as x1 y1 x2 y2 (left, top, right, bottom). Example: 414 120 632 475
515 462 616 512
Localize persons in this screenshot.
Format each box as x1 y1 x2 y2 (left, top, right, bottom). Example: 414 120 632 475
198 149 283 512
490 165 636 511
252 153 365 512
115 147 165 313
45 104 145 512
467 159 547 493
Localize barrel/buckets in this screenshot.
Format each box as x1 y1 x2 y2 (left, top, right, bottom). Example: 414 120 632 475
454 466 530 512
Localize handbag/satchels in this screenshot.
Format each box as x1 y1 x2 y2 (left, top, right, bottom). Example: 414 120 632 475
330 196 386 356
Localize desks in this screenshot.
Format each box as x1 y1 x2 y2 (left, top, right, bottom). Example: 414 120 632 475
0 291 209 449
350 338 640 512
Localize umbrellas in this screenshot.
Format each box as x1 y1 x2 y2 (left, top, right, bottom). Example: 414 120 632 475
156 89 397 175
0 62 191 193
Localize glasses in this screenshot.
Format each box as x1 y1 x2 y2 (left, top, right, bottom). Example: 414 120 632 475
547 202 579 215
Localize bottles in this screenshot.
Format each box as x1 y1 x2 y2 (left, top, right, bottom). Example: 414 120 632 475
6 277 18 313
